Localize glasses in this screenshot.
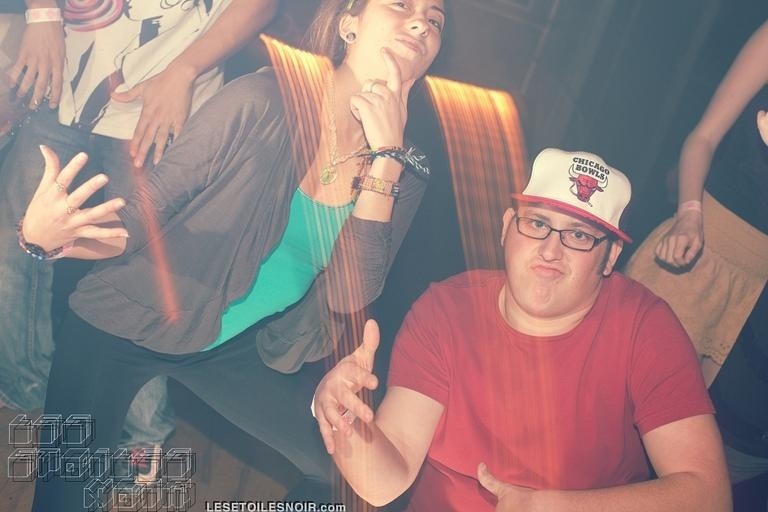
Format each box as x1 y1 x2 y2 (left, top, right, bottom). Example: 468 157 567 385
515 211 609 252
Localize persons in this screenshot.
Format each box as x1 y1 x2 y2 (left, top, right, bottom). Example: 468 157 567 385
0 1 282 511
620 18 768 388
308 145 738 512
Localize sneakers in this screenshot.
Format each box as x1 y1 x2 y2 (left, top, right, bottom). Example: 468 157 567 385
120 445 161 486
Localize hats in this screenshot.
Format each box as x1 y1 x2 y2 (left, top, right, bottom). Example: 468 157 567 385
510 148 633 244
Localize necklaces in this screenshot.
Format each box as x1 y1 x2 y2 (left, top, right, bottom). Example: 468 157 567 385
318 101 368 185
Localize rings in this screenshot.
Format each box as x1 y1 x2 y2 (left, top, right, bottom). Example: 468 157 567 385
54 179 68 194
65 198 80 215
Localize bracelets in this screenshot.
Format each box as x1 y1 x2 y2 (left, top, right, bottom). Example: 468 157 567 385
16 213 76 263
674 198 703 215
350 174 402 199
14 1 446 511
19 6 65 24
309 388 359 426
